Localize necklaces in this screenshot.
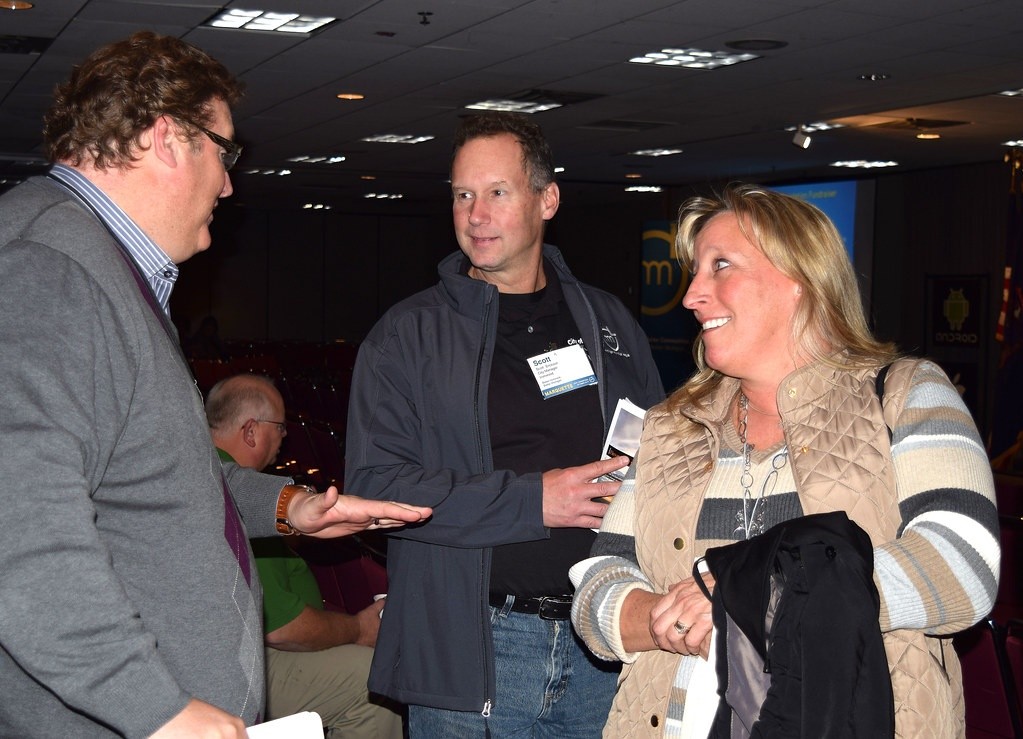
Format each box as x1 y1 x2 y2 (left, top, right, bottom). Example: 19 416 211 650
738 395 792 538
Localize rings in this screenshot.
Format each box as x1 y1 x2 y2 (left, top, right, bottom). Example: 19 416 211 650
375 517 380 525
674 621 689 635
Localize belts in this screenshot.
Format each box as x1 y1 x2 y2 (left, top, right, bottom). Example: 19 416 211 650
488 590 572 621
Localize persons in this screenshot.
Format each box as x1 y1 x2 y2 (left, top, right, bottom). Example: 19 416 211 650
0 33 432 739
568 189 1004 738
206 374 379 651
173 313 225 358
344 121 666 739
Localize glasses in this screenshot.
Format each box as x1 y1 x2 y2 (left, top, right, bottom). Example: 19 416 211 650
256 419 286 431
177 114 244 171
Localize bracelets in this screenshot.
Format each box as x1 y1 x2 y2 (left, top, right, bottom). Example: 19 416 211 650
276 484 312 535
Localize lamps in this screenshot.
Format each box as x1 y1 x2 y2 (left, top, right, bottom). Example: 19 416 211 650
792 120 812 149
